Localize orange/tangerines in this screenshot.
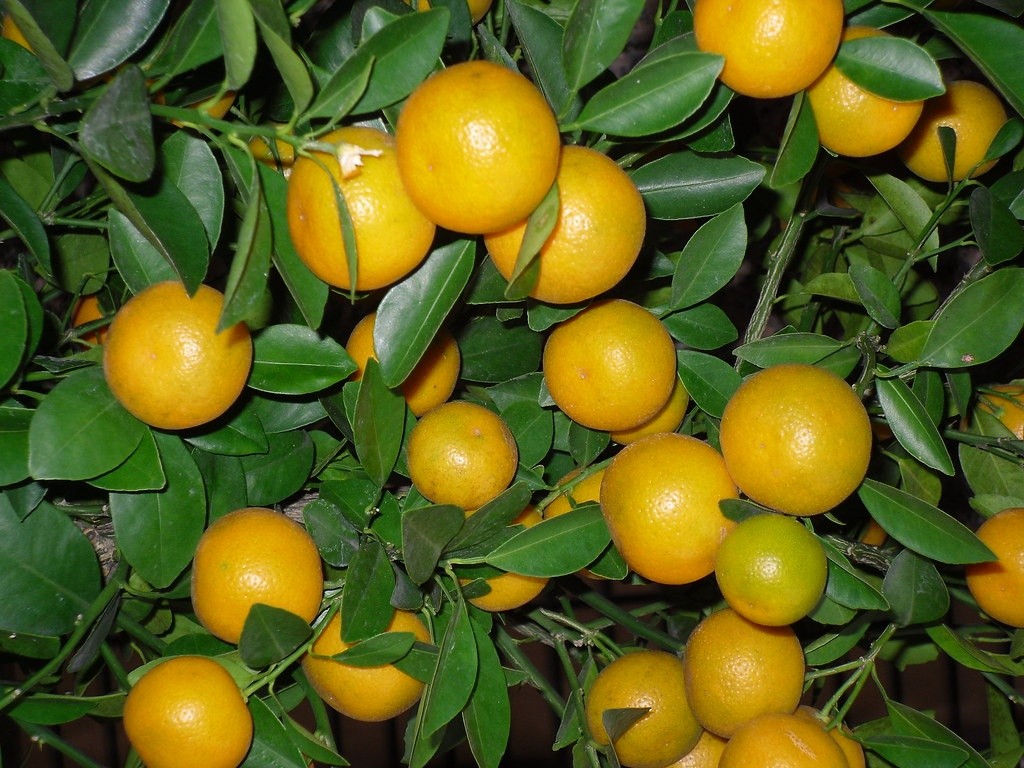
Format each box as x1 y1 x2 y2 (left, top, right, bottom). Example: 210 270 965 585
74 1 1024 768
